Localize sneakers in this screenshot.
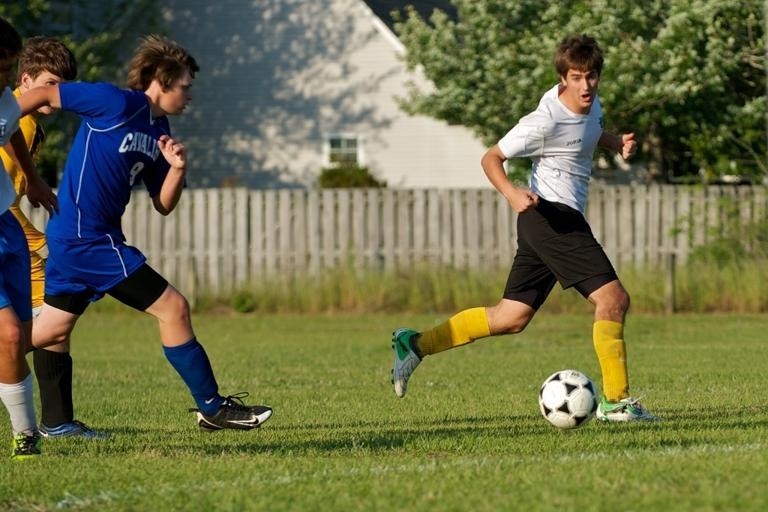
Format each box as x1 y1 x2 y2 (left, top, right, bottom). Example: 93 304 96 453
391 329 423 397
197 396 272 432
9 420 108 462
594 395 659 423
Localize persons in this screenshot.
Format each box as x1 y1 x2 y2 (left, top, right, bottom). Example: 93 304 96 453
1 18 78 462
0 33 273 432
391 34 665 423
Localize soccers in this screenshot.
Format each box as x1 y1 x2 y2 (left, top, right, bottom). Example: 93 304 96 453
539 370 598 428
